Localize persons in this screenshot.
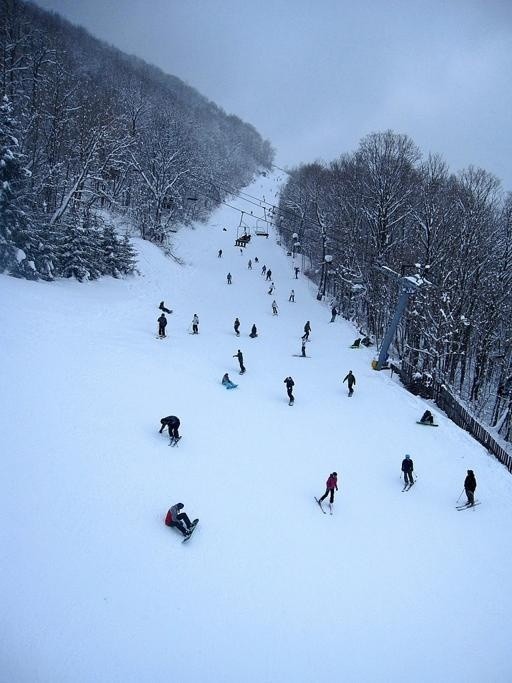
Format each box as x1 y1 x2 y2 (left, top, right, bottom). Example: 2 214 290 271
420 410 434 425
342 370 356 395
158 415 181 441
222 373 238 390
217 171 391 370
157 313 167 338
192 313 200 334
164 502 196 536
158 301 172 314
233 349 249 373
463 470 477 508
401 454 414 485
318 471 339 507
284 376 295 403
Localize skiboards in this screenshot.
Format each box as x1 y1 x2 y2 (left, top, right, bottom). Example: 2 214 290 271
401 474 417 493
315 496 333 515
167 436 182 447
456 500 482 513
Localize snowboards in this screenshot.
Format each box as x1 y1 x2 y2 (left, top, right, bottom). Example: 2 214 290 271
292 354 310 358
182 519 199 544
416 421 437 427
239 368 246 375
236 333 240 337
226 384 238 390
348 390 354 397
289 397 294 406
